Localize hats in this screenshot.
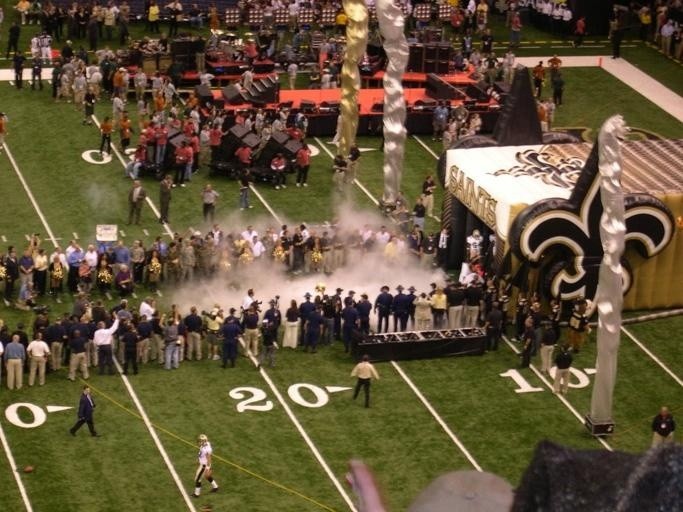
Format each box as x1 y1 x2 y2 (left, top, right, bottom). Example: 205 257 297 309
336 288 368 305
380 279 477 294
119 66 127 72
303 292 312 298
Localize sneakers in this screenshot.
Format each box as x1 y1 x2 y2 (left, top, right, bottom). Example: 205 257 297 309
511 338 520 343
274 182 308 190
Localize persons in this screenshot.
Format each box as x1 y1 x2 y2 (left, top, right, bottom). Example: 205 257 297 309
652 407 673 447
190 430 220 499
69 386 102 439
0 1 683 406
